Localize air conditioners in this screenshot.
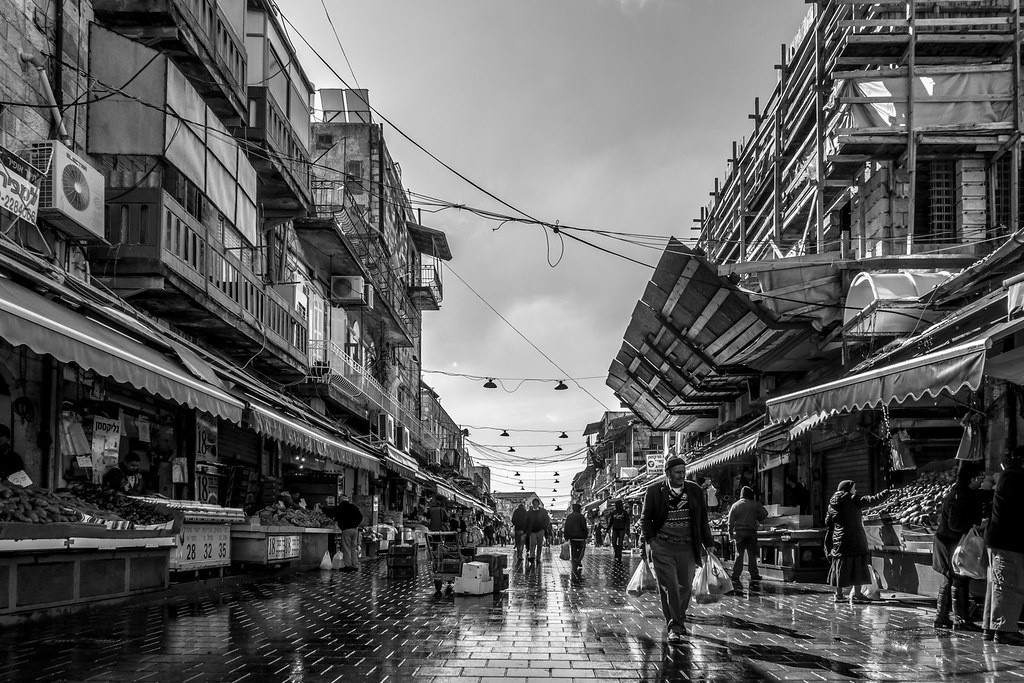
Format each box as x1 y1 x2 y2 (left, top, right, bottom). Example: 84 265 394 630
343 284 374 310
378 413 394 445
27 140 105 239
397 426 409 452
430 448 441 464
331 276 365 300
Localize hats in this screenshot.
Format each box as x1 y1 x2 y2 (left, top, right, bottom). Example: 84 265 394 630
664 456 686 471
339 493 348 501
838 480 854 493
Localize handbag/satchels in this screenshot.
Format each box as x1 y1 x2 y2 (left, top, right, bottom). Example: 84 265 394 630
320 550 332 569
441 523 450 531
559 540 570 560
848 565 880 600
331 551 345 571
626 558 658 598
692 552 734 604
952 526 987 580
603 533 610 547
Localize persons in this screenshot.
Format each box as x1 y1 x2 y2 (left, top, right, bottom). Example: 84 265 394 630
824 480 898 604
564 504 587 568
483 521 507 547
728 486 768 582
982 445 1024 645
640 453 715 641
931 463 994 629
606 500 630 560
320 495 363 571
449 516 468 546
512 499 550 560
107 452 143 495
426 500 447 550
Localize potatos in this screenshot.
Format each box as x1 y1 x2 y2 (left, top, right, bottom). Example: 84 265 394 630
0 479 80 523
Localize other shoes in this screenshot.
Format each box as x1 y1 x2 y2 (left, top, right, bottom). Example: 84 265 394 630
750 572 763 579
982 630 995 641
852 593 874 604
678 624 687 635
731 573 741 585
667 631 680 643
342 567 358 572
953 622 983 631
993 629 1024 644
528 556 534 563
576 559 583 567
933 615 955 629
536 560 541 564
835 595 848 603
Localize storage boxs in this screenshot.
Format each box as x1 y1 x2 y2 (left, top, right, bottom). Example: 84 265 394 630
388 535 508 596
235 467 281 508
762 505 813 529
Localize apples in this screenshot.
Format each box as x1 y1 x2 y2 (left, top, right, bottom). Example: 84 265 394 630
866 468 957 527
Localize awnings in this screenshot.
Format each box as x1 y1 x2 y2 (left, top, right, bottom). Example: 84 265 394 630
645 424 790 485
422 477 494 517
229 390 381 474
353 441 416 480
765 314 1024 440
0 275 244 426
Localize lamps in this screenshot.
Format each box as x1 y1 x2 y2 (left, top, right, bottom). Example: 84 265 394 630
554 381 568 390
514 472 525 490
483 380 497 389
500 430 509 436
508 447 515 452
551 472 559 506
558 432 568 438
555 446 562 451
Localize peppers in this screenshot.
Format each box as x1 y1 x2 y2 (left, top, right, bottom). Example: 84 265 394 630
70 481 173 525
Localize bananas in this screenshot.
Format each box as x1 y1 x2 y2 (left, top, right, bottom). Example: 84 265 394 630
259 504 336 528
